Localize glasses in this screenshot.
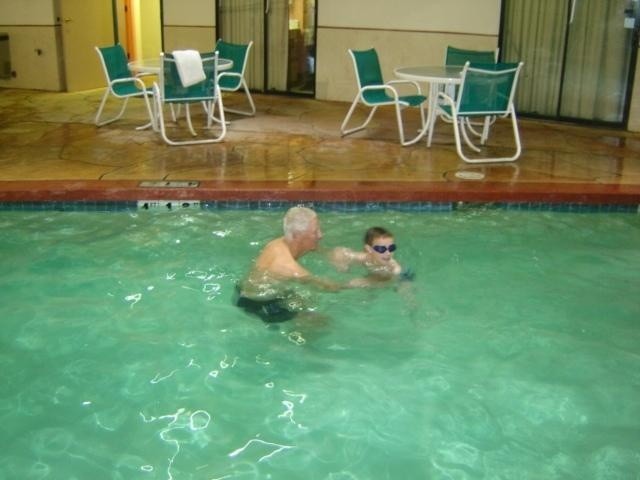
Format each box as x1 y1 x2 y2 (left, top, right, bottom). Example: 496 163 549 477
371 244 396 254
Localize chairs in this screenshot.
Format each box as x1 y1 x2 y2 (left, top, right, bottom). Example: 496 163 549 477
92 38 255 148
339 45 525 164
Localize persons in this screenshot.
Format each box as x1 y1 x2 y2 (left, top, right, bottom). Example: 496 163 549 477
232 205 353 336
341 225 415 293
